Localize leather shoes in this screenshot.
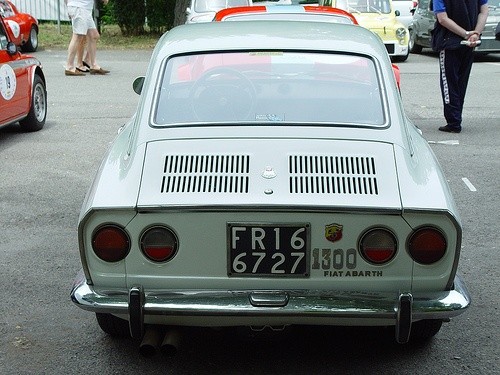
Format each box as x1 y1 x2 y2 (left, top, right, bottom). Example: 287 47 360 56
439 125 461 133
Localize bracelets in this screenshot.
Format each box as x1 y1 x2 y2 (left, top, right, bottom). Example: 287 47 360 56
464 31 470 39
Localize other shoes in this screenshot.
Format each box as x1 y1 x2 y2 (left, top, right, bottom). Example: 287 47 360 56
83 61 90 68
65 69 86 76
90 67 110 74
76 67 89 72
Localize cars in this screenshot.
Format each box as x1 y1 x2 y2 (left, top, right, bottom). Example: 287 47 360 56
0 0 39 52
0 14 47 130
69 0 471 344
409 0 500 53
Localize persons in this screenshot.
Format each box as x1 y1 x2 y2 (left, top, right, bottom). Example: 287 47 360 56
76 4 101 72
64 0 110 76
431 0 489 134
